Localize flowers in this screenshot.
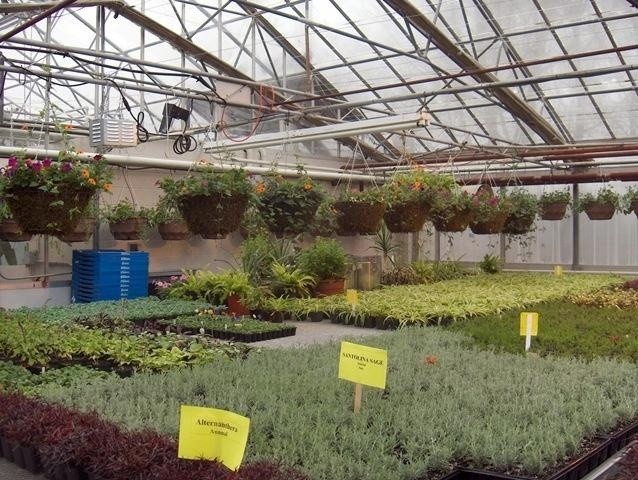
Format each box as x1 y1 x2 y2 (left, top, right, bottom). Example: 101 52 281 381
1 142 637 241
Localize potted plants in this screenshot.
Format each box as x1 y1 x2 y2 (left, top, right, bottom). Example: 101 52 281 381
1 233 477 391
